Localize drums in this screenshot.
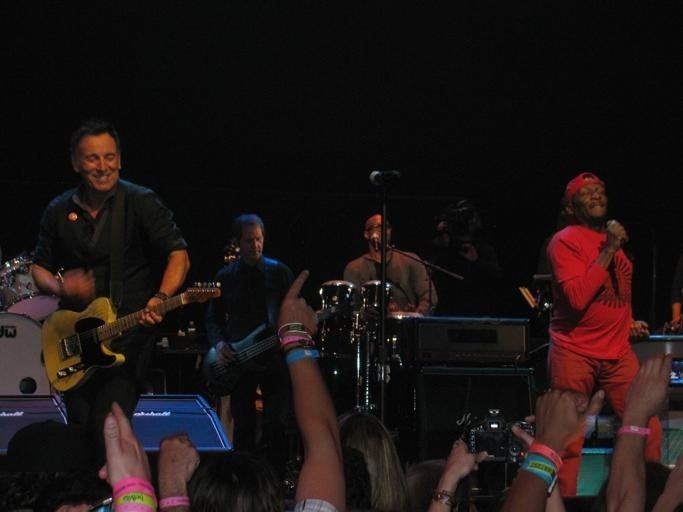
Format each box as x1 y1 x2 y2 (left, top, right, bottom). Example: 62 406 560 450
361 279 392 303
631 335 683 387
319 280 356 305
0 256 60 397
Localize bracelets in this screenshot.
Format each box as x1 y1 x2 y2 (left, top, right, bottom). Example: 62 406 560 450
278 323 320 366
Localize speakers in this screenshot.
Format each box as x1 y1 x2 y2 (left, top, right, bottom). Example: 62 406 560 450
0 395 69 454
134 395 235 457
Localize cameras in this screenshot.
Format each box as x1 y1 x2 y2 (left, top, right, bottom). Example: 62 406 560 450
669 357 683 387
463 409 536 465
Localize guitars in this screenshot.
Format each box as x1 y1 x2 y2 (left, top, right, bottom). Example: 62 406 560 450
200 306 339 398
41 281 221 392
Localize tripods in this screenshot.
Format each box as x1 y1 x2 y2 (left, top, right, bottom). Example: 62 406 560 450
321 305 406 418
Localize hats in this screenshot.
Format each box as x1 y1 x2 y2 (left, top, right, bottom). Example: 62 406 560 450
564 172 604 202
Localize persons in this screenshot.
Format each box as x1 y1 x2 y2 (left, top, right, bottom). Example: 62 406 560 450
276 269 347 512
341 213 440 436
2 402 489 512
204 213 297 452
31 118 191 461
543 171 664 502
499 355 682 512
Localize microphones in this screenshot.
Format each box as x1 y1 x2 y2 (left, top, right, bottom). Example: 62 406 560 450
372 234 381 251
371 169 400 182
606 221 625 244
